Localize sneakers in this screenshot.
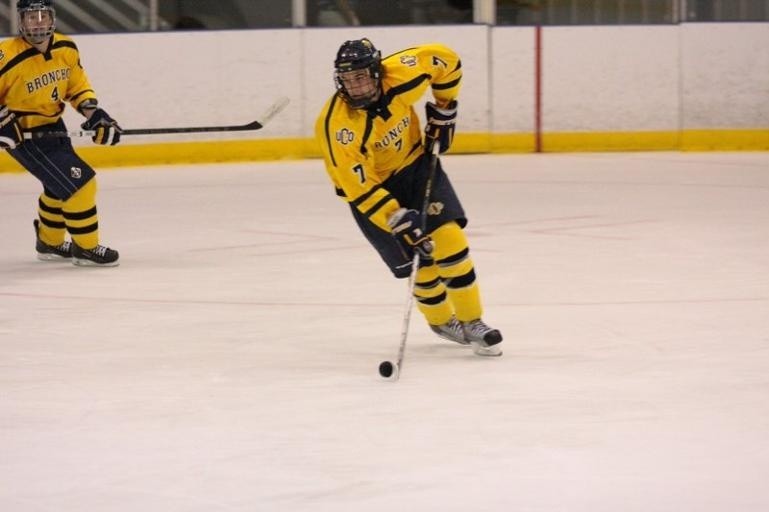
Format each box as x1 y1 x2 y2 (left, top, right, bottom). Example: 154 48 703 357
67 241 120 264
430 312 470 347
461 319 502 348
33 239 71 257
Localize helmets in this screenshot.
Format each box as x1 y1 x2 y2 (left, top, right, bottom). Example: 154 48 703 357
17 0 57 45
333 38 385 111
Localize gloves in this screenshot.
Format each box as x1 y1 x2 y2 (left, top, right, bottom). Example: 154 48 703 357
0 105 22 151
388 206 434 267
80 106 123 147
423 102 458 153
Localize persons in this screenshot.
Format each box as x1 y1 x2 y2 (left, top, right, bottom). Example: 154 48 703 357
312 35 504 349
1 1 124 264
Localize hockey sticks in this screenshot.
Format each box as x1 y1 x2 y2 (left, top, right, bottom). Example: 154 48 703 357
23 96 290 139
380 140 439 383
